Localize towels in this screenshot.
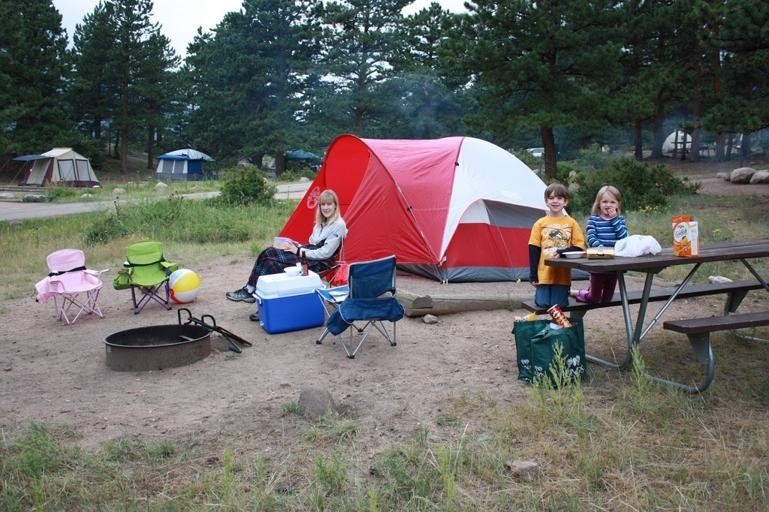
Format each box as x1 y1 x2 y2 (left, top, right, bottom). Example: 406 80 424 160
614 235 665 257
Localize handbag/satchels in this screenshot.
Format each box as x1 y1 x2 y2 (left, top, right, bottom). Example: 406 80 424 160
512 316 587 387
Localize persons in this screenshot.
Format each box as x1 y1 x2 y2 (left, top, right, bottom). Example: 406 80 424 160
569 185 628 304
226 189 346 320
528 182 586 310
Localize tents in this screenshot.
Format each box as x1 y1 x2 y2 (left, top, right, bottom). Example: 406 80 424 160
284 150 321 172
153 147 216 181
272 134 591 283
12 146 101 189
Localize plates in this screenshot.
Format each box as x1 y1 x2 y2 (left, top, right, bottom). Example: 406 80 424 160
561 250 586 259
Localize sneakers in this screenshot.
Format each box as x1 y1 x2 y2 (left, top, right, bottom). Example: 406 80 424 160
249 310 259 320
226 287 255 303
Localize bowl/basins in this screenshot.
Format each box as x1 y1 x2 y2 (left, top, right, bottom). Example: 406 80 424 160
274 235 291 250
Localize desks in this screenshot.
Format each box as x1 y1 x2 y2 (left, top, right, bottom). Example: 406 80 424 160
545 240 769 394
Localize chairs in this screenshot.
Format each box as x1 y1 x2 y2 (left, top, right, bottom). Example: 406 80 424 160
114 241 179 314
314 254 404 358
35 249 111 325
317 228 348 286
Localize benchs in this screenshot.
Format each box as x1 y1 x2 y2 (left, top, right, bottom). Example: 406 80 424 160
663 311 769 335
521 280 769 316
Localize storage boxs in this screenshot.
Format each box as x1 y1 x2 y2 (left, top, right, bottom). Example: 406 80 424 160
252 270 324 334
